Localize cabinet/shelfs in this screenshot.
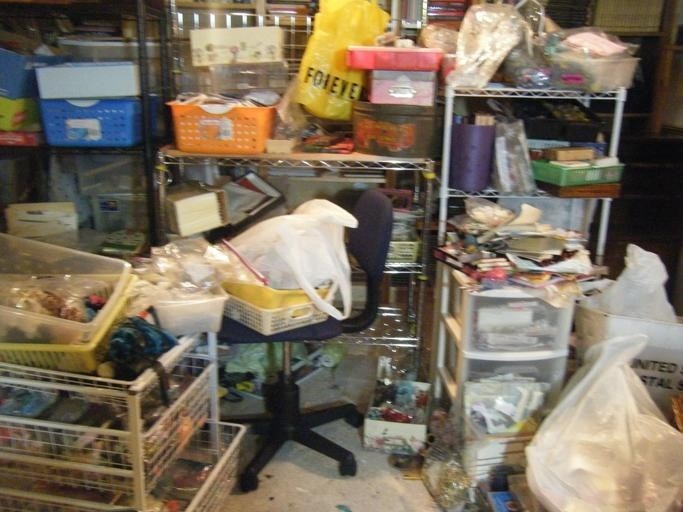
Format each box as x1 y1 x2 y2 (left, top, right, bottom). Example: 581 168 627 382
161 139 435 385
433 82 627 287
1 0 172 259
434 282 573 407
4 329 245 512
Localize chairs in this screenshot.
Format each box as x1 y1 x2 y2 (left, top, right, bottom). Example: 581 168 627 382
203 189 392 494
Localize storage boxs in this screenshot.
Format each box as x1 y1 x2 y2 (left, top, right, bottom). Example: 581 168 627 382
364 376 432 456
370 70 435 109
352 99 440 158
576 304 681 427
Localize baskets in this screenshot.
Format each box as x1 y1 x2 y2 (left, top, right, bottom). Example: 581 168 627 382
0 333 203 408
165 98 281 155
0 273 139 373
224 293 335 336
526 139 627 186
33 94 159 148
0 352 215 496
0 421 247 512
348 230 422 265
